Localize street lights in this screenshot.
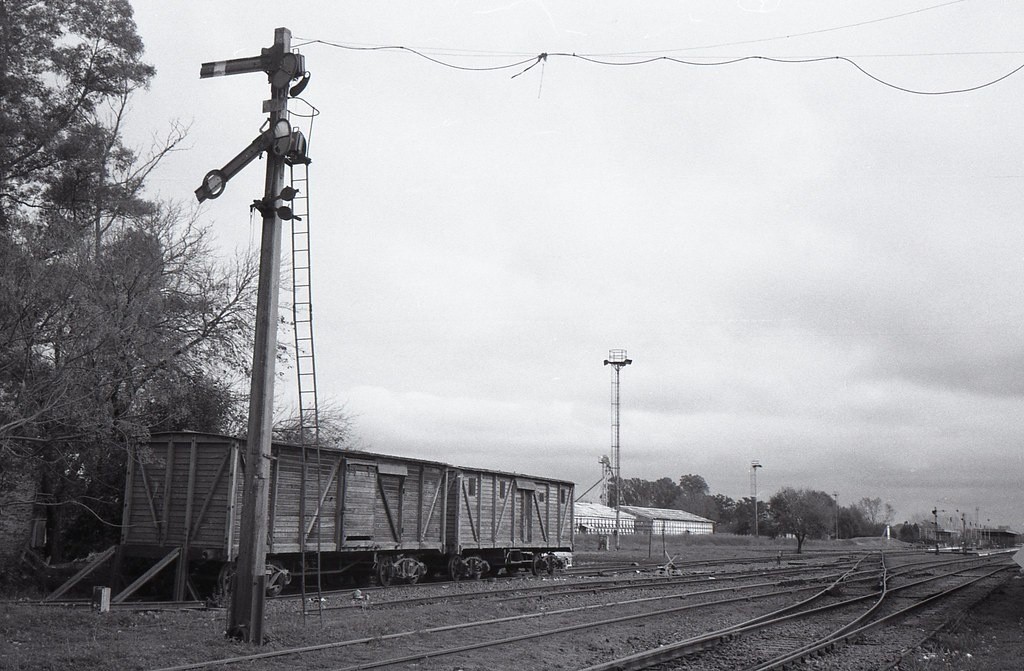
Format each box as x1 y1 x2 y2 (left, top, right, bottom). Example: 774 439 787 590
603 349 632 550
752 461 762 536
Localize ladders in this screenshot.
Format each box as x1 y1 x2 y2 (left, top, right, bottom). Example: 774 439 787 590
285 159 327 627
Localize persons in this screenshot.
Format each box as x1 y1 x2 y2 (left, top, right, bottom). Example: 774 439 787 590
776 550 782 565
